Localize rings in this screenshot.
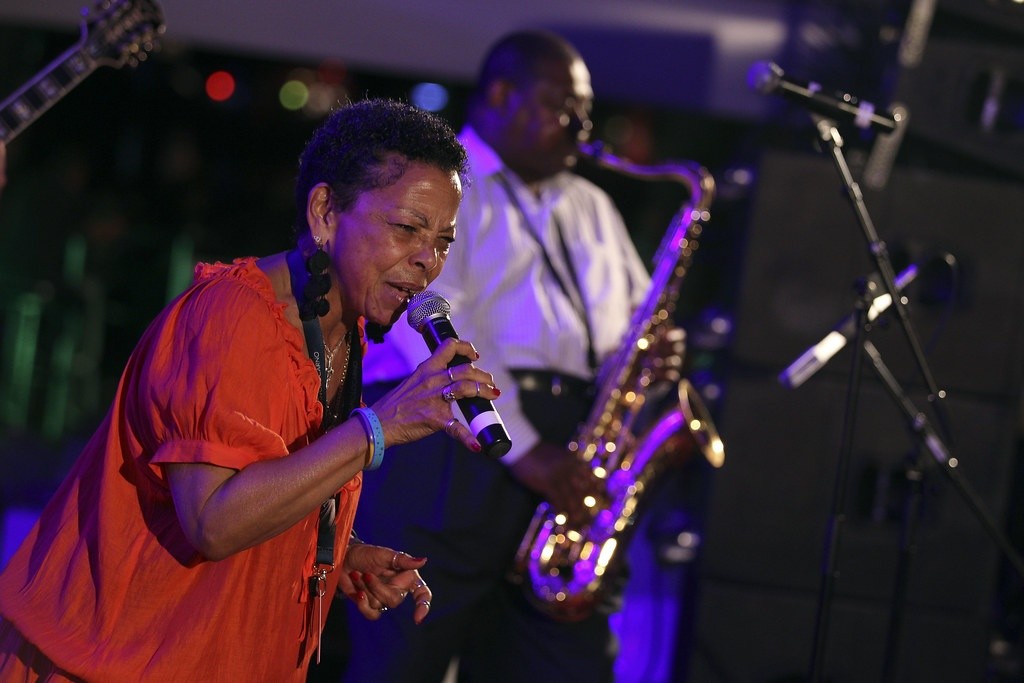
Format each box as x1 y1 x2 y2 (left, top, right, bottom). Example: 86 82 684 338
379 604 389 612
401 591 405 603
448 367 456 383
475 381 481 397
446 417 460 435
415 600 431 608
392 551 405 571
441 385 456 403
411 578 426 593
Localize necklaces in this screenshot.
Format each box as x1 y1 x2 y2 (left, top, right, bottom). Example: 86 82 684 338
282 241 363 663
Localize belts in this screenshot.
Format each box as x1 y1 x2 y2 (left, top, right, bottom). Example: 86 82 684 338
503 370 583 407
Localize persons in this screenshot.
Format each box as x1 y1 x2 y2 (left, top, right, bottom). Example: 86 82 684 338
346 33 683 683
0 99 501 682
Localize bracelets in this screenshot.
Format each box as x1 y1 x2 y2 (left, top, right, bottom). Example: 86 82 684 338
348 405 385 472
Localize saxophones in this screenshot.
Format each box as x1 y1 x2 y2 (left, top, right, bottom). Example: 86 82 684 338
506 143 725 621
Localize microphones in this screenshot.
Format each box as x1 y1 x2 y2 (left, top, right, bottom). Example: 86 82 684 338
742 63 895 136
405 291 513 457
774 269 922 388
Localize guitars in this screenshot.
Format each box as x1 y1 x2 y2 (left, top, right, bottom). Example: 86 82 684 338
0 0 167 147
